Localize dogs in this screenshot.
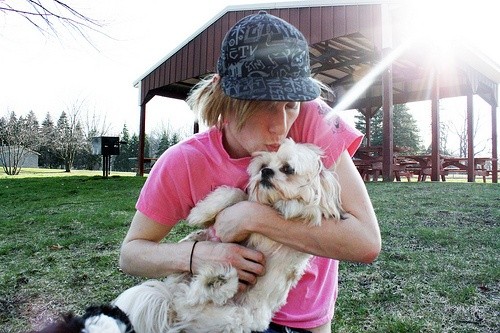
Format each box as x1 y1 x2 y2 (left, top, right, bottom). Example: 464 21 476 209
81 135 342 333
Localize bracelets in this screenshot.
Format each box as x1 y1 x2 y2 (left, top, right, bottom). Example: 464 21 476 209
189 241 199 274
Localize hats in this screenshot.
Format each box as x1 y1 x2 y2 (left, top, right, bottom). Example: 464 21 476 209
218 9 321 101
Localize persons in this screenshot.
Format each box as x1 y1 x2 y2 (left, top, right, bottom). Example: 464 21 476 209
118 10 382 332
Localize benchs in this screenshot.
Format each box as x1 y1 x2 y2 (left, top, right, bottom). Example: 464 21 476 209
351 144 498 183
